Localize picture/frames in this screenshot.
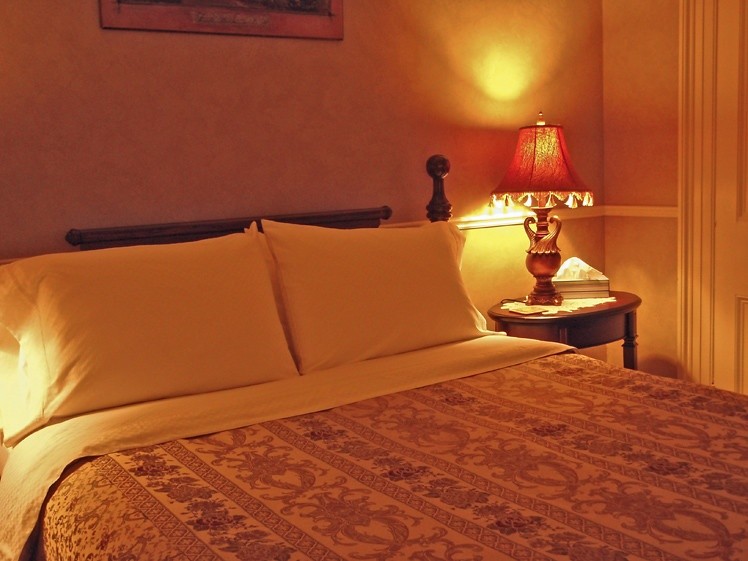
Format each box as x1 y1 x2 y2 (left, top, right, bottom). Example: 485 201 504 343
97 0 345 42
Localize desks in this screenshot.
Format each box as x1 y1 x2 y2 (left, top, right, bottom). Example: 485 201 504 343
486 289 642 372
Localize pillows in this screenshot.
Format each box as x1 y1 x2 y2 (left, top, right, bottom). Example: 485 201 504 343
259 219 508 374
0 222 297 447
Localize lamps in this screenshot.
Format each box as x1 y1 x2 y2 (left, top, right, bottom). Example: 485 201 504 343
484 111 595 306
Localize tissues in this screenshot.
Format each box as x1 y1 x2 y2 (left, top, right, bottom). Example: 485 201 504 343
551 256 610 299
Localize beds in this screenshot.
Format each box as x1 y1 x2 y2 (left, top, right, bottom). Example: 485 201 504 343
35 153 748 561
